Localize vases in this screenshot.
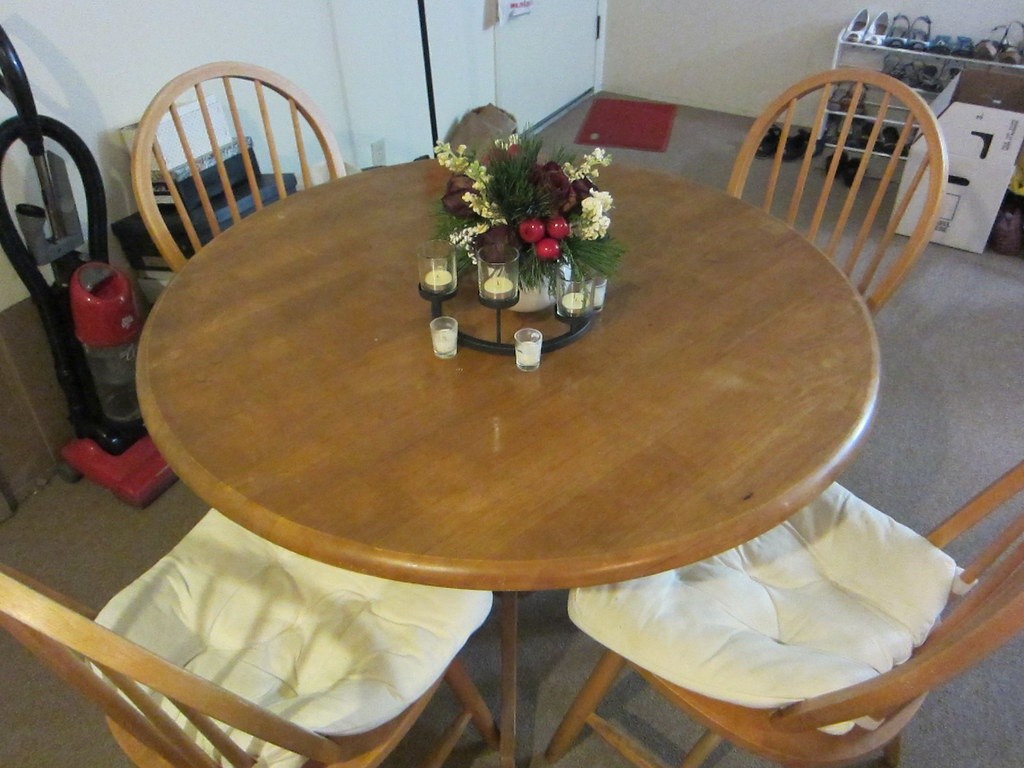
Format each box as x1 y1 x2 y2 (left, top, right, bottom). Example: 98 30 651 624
487 254 573 312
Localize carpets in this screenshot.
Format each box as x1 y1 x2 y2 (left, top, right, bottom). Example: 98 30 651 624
575 98 677 151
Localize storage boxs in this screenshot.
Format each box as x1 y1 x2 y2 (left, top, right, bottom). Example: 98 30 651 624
887 68 1024 255
111 173 298 279
151 137 257 215
119 92 228 168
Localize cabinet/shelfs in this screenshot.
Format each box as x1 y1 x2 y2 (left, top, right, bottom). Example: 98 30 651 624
810 28 1024 184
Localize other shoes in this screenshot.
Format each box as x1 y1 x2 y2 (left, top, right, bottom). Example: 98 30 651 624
827 82 855 110
840 81 868 114
879 124 909 155
825 151 845 176
859 121 885 152
879 53 963 93
751 123 783 159
781 129 811 160
837 118 857 147
842 156 861 187
824 113 841 145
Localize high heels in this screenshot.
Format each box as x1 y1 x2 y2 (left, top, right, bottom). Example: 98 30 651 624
841 8 1024 65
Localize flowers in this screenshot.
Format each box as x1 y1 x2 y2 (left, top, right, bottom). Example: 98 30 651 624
421 121 630 301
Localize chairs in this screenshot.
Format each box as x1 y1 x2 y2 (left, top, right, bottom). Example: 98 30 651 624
131 62 347 272
0 555 501 768
726 67 949 319
542 462 1024 768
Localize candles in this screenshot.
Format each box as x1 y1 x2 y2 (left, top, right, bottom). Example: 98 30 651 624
516 342 541 364
562 293 589 313
483 277 513 298
433 330 457 352
424 270 453 289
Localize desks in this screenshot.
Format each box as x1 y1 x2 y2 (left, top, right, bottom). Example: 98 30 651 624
135 156 882 768
